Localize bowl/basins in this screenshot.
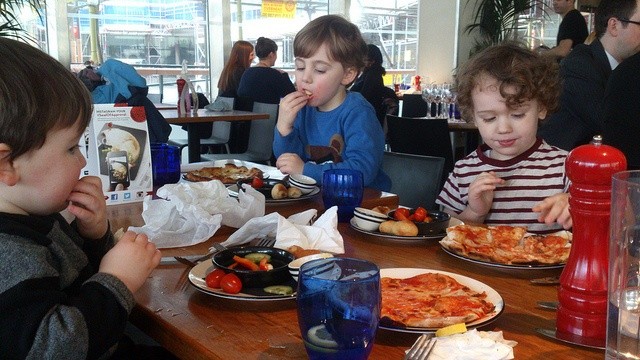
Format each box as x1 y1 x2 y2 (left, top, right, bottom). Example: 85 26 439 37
212 247 297 285
235 178 289 198
288 173 317 195
387 208 451 236
353 207 388 232
288 253 335 282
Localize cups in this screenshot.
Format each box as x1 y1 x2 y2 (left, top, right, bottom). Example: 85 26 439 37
296 257 381 360
321 168 364 222
605 170 640 360
150 143 190 187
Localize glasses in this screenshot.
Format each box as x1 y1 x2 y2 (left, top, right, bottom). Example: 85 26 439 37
616 19 640 27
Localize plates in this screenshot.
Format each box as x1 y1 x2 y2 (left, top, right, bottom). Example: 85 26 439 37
439 229 572 270
188 246 342 302
349 208 465 243
182 172 270 184
328 268 504 333
225 184 320 205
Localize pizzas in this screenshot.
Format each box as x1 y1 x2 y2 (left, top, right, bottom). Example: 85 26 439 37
181 161 265 186
439 220 576 266
338 272 495 330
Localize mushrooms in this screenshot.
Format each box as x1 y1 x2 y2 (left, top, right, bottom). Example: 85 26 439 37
271 181 288 200
392 219 419 237
378 218 395 234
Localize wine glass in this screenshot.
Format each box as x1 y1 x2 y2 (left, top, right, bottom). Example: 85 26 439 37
393 74 402 93
422 80 459 123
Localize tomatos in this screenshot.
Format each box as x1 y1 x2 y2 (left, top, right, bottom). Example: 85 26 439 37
205 268 225 289
219 273 243 294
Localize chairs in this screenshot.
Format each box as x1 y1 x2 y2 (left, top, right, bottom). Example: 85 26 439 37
199 100 279 160
369 150 445 211
148 93 162 103
386 114 454 174
168 96 235 156
403 94 428 116
183 94 212 138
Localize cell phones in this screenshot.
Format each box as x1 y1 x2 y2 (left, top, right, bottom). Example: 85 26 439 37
106 151 131 188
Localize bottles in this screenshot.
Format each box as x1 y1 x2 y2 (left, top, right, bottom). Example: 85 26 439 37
176 59 191 115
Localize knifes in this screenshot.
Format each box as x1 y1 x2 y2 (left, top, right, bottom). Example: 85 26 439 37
535 326 639 360
535 300 557 311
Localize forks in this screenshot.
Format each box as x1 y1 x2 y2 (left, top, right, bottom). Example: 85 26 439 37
213 241 229 253
254 237 276 249
174 232 260 265
403 334 437 360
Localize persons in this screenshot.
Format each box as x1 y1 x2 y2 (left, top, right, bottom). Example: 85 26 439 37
537 1 640 170
272 15 393 193
217 40 256 110
0 37 178 359
236 37 297 154
434 44 574 232
540 1 589 57
350 44 386 101
